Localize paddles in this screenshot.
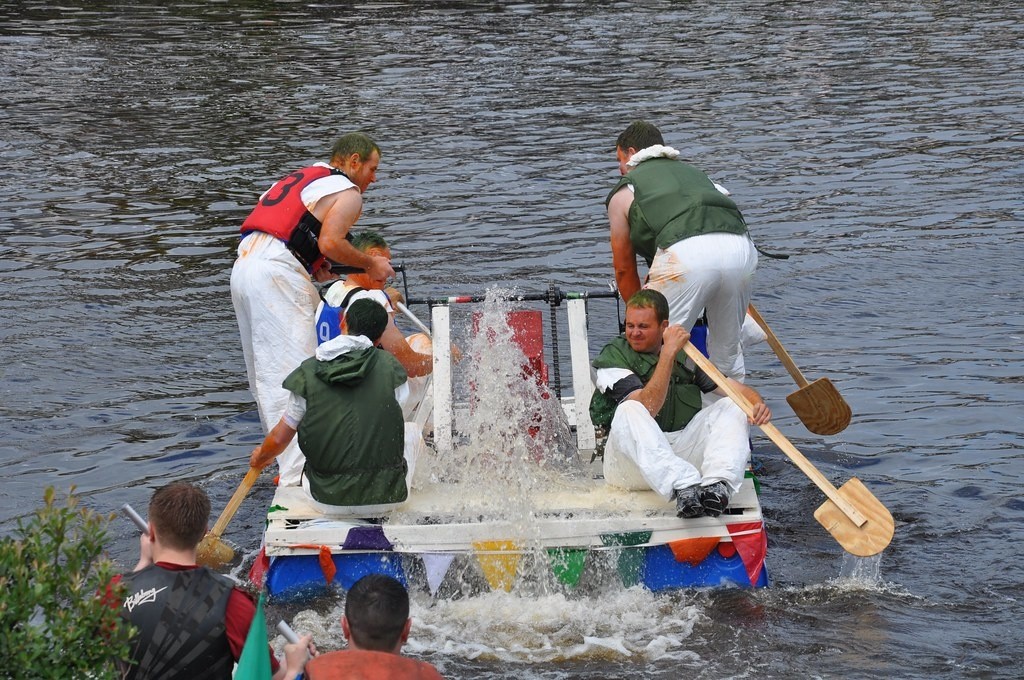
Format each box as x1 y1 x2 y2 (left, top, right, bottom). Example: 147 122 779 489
682 301 894 558
194 466 266 569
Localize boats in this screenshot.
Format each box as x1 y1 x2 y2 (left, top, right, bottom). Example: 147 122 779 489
256 283 772 610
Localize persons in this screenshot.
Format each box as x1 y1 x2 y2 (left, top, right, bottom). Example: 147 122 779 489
284 570 453 679
582 290 773 520
247 296 414 524
223 131 397 488
599 123 757 404
681 282 768 375
91 481 310 680
314 230 444 380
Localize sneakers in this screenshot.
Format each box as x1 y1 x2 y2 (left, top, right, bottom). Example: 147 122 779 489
702 481 730 516
677 486 701 518
